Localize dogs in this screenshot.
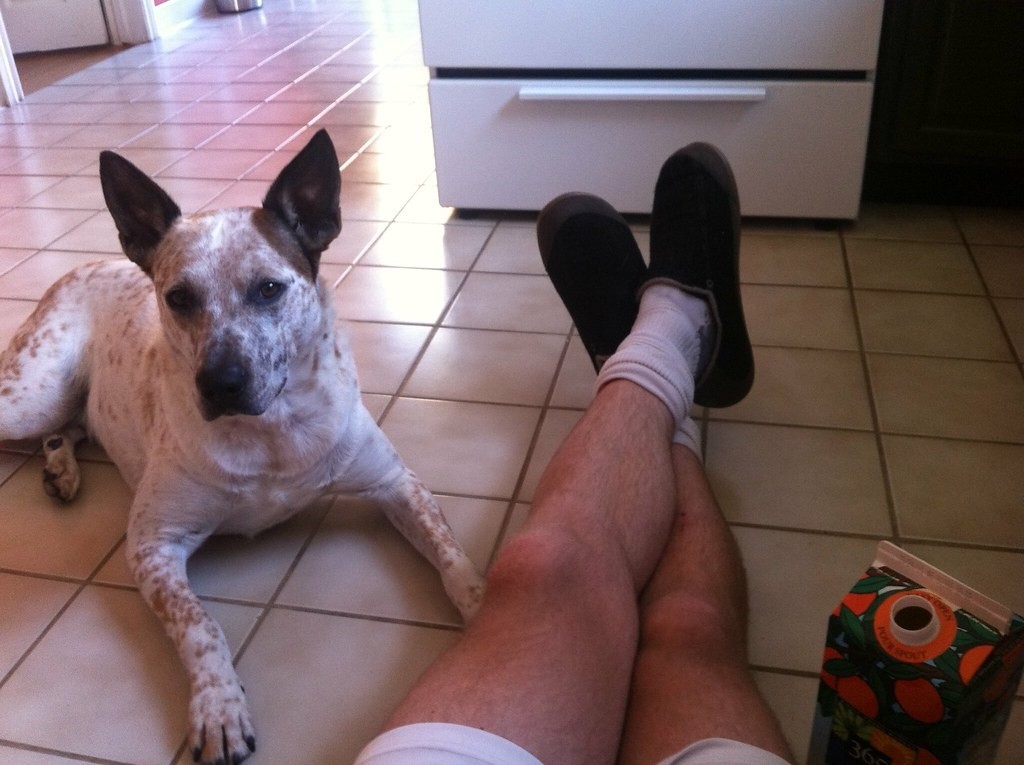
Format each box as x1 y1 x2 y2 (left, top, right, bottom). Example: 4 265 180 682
0 128 486 764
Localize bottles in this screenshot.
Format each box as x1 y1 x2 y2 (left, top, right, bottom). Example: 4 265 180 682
806 539 1023 765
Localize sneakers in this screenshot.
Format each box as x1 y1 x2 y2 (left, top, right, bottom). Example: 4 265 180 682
537 191 650 374
636 140 755 409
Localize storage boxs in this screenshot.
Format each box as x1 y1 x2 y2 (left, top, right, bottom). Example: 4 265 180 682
419 1 886 225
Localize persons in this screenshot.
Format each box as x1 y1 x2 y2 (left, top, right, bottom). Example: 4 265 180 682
355 142 803 765
